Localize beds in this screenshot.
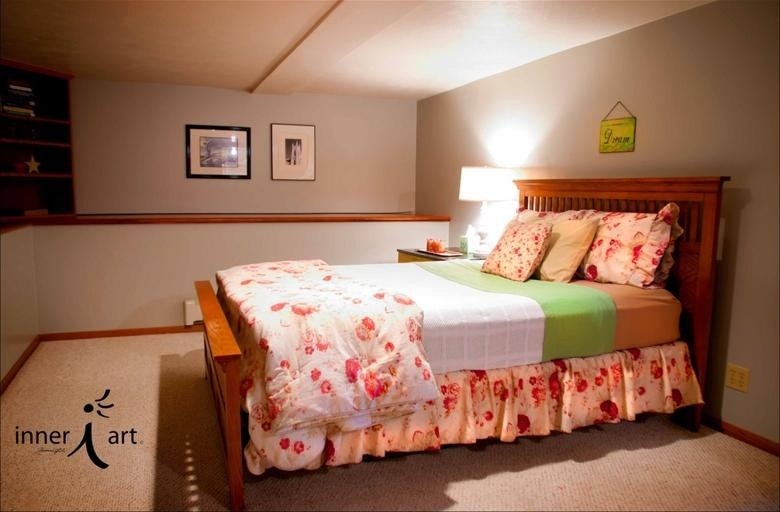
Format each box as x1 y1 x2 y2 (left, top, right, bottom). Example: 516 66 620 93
193 174 730 512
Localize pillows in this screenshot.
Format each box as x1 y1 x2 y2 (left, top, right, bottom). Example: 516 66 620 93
480 199 690 294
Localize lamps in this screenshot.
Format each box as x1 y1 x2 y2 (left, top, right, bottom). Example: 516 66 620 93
459 165 507 260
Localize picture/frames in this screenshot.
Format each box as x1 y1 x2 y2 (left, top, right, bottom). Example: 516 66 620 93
185 120 317 183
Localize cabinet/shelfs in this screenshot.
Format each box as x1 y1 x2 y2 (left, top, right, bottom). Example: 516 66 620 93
1 59 75 223
